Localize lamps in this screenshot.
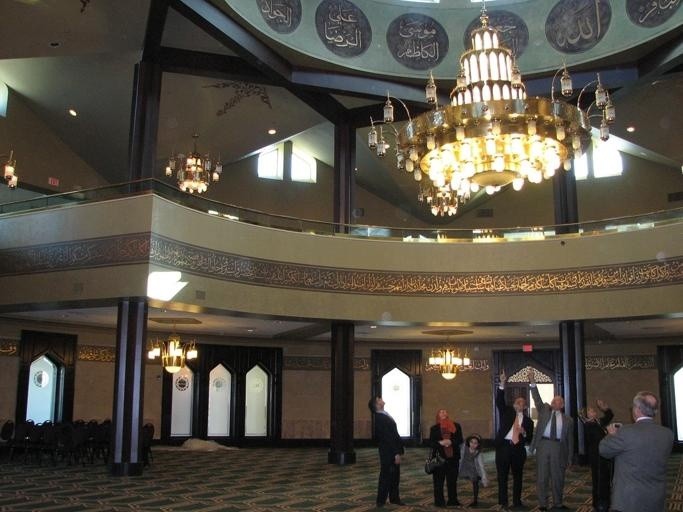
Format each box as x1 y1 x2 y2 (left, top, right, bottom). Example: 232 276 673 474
0 149 18 190
146 320 198 375
423 334 471 381
164 129 224 194
367 1 616 218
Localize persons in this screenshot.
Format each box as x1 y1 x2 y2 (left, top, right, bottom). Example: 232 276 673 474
368 396 404 512
495 370 533 512
458 433 487 508
527 368 574 512
430 409 463 509
578 400 614 512
598 391 675 512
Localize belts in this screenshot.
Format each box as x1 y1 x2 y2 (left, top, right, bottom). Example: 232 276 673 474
541 436 560 441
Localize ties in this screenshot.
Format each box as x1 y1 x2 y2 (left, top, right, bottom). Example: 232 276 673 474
550 411 556 441
511 414 520 445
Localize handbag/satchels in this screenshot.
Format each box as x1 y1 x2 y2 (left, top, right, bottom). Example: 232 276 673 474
424 456 448 474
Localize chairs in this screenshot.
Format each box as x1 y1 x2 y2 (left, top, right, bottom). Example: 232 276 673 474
0 418 155 471
